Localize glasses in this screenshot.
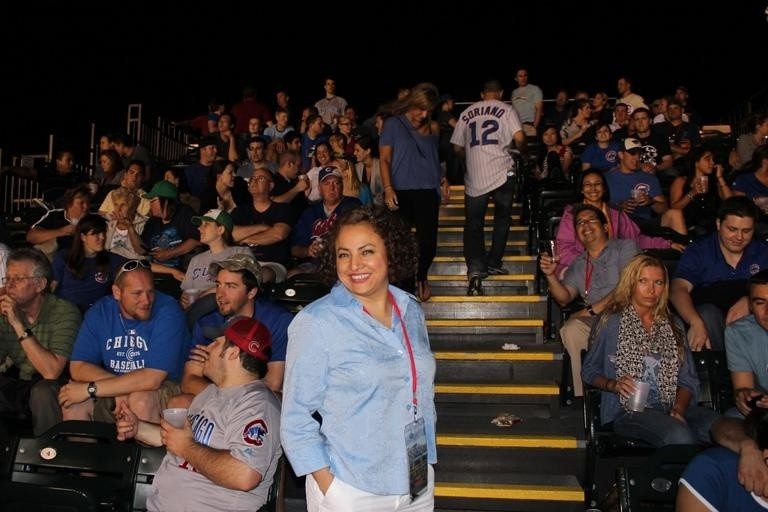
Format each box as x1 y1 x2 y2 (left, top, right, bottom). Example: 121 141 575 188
250 175 269 182
1 277 35 284
114 259 151 281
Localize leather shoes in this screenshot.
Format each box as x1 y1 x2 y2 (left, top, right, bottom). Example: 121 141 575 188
487 267 509 275
468 278 483 295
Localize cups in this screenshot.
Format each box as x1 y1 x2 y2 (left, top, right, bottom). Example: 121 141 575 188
162 407 188 429
630 190 641 199
185 288 200 305
544 240 561 264
627 380 650 413
696 176 709 193
71 218 79 225
298 175 307 180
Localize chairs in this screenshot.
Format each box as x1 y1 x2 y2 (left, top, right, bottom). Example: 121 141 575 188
0 419 286 512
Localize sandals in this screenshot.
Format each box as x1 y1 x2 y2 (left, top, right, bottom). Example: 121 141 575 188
418 280 430 304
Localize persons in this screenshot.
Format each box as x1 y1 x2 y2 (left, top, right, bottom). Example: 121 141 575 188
512 72 768 404
725 269 767 421
58 258 191 445
1 82 452 327
452 76 528 293
115 316 283 512
279 204 438 511
676 407 768 512
581 254 724 457
168 254 294 410
0 246 82 472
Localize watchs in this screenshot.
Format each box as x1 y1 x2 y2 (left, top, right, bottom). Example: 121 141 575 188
16 328 34 342
86 382 97 398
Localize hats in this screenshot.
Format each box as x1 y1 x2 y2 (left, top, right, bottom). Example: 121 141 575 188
142 180 178 199
191 209 234 231
209 254 263 286
319 166 342 182
202 315 272 362
619 138 646 152
